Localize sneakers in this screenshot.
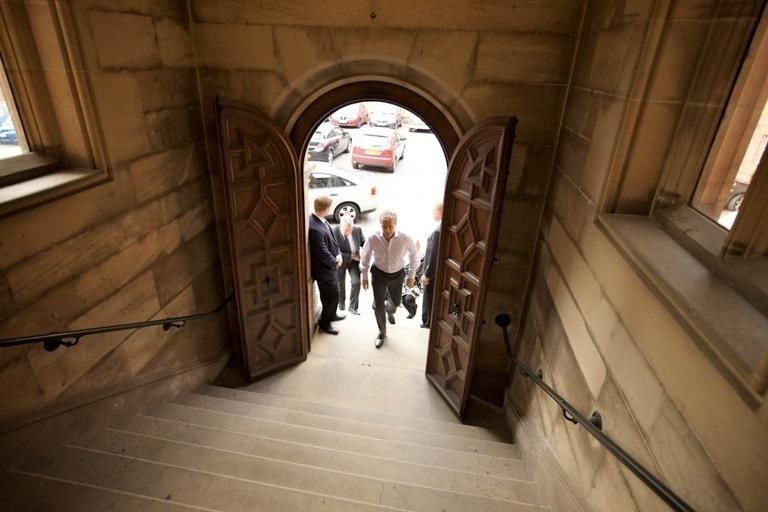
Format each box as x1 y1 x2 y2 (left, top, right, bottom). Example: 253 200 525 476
326 304 360 334
375 333 385 347
388 313 395 324
420 324 425 328
407 313 414 318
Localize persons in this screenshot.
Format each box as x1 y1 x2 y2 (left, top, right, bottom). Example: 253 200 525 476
332 213 366 315
401 239 424 319
309 196 346 335
420 202 444 328
358 209 419 348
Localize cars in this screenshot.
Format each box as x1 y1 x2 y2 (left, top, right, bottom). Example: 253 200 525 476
305 170 379 224
305 101 432 173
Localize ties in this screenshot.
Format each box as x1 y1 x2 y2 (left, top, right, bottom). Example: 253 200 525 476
344 235 351 256
